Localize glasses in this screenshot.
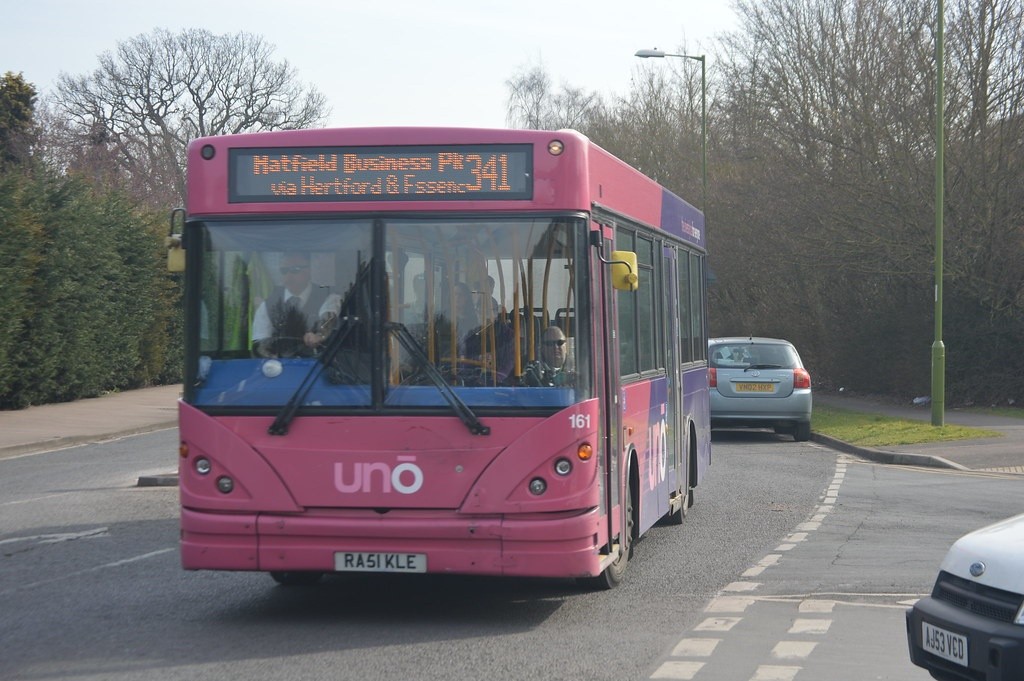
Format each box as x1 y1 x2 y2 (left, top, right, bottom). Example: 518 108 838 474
544 340 565 346
280 266 307 273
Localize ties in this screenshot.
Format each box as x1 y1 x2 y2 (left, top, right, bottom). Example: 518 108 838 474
289 298 299 315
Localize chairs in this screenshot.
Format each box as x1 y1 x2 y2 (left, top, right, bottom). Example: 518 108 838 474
498 307 576 360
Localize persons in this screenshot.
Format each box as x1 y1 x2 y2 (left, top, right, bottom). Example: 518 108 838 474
537 326 574 376
394 273 427 326
477 274 498 323
251 251 343 359
465 296 517 386
441 280 477 334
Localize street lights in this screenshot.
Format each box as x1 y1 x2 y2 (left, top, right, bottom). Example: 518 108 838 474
634 46 708 211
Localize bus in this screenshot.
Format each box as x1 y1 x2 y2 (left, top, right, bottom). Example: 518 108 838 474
164 126 714 592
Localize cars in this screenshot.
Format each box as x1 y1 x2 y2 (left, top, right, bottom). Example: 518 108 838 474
705 336 815 442
900 510 1024 681
